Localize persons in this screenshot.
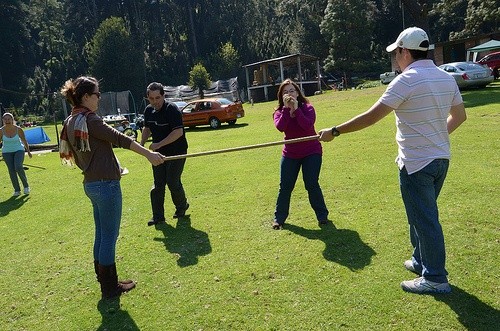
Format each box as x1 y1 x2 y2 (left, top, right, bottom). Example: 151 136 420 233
272 79 329 230
140 83 188 226
318 27 466 293
0 113 32 197
60 75 165 299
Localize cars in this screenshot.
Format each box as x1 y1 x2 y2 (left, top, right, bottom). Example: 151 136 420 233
135 114 152 137
478 52 500 79
438 62 494 89
181 98 244 129
172 102 188 110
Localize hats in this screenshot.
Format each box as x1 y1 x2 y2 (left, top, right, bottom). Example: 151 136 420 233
386 27 430 52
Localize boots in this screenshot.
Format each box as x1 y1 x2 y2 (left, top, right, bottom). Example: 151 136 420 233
94 261 136 298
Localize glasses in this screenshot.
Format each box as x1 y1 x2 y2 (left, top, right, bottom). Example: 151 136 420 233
282 90 297 95
88 92 101 99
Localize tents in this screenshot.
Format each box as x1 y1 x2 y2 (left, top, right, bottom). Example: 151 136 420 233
466 40 500 62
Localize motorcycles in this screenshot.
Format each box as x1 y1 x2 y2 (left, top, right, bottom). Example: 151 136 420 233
110 108 138 141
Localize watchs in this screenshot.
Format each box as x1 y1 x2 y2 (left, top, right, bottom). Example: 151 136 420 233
331 126 340 137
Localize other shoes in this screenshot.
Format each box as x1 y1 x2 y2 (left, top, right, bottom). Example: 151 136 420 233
24 188 29 194
273 221 281 229
173 203 189 218
148 216 165 226
400 277 451 294
319 221 327 226
12 192 21 197
404 260 420 275
